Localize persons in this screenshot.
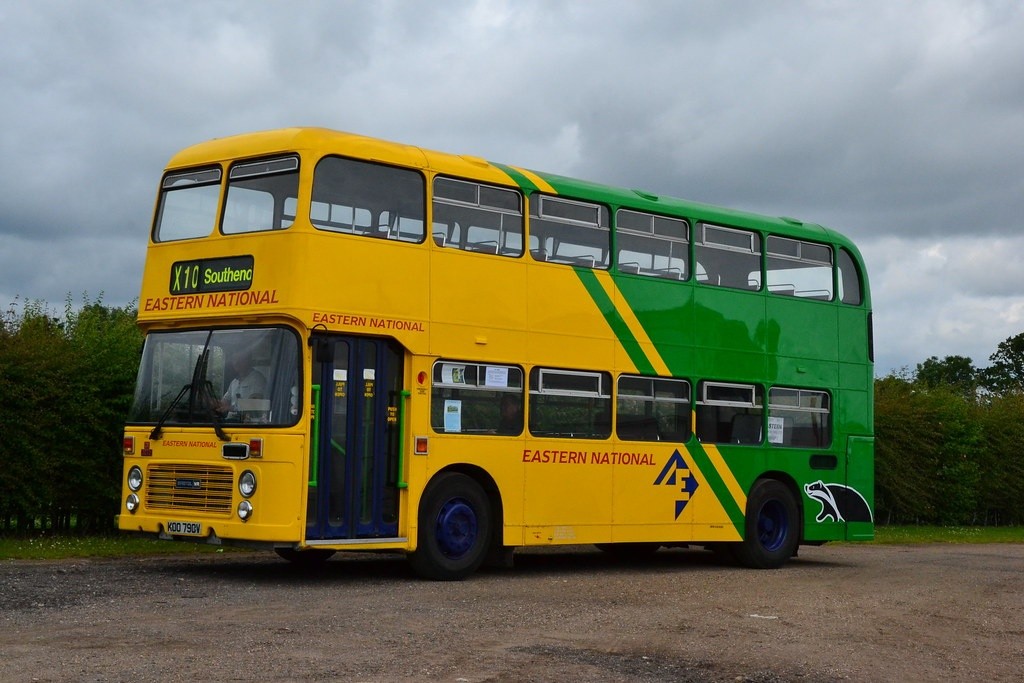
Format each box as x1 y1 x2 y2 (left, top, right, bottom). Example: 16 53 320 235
490 394 520 436
211 343 266 417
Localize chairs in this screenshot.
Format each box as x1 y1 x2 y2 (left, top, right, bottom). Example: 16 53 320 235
247 365 271 399
362 224 831 301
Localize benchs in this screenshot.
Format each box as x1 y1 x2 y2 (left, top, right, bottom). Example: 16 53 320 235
530 420 831 449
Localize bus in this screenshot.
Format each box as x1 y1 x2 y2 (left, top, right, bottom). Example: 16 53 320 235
113 126 874 582
